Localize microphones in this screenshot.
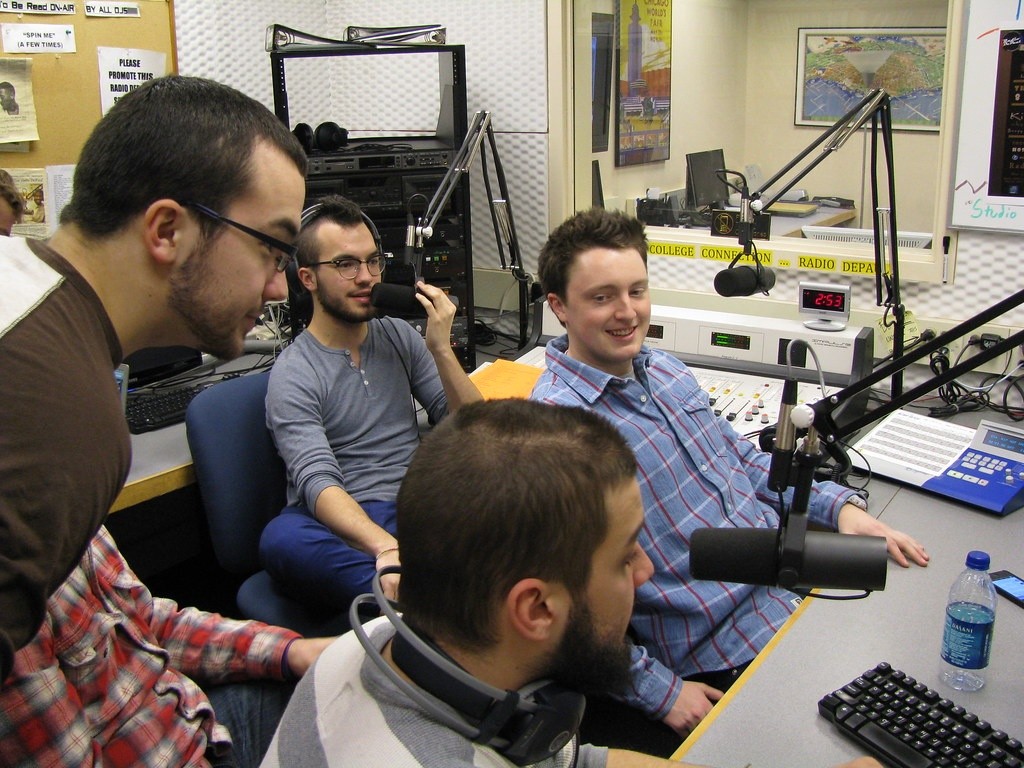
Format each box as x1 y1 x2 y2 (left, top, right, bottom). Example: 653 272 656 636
715 265 776 297
369 282 461 317
689 527 887 591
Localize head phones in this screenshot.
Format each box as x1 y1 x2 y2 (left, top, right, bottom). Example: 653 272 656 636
293 121 349 154
350 565 586 765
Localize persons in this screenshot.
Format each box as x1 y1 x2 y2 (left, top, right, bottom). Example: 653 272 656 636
260 397 884 768
529 202 929 737
0 82 19 115
0 524 337 768
0 77 310 680
29 190 44 223
0 170 25 236
257 192 483 610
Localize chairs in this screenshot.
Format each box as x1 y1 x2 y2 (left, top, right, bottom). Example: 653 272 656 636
185 371 339 638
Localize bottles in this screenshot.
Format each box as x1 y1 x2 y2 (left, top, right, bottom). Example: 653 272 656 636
940 550 998 690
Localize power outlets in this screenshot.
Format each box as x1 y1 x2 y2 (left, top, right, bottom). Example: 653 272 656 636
961 325 1009 374
914 318 964 369
1006 327 1024 378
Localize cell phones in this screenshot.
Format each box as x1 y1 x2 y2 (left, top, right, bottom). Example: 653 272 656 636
989 570 1024 609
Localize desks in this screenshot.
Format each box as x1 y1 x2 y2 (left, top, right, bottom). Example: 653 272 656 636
107 309 1024 768
664 206 856 237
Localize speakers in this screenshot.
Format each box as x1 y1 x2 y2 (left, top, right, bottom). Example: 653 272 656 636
667 195 680 228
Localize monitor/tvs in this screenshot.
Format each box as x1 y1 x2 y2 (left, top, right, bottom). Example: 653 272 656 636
123 346 203 392
687 150 729 215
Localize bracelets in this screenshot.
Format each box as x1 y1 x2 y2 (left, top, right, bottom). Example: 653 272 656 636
376 547 398 558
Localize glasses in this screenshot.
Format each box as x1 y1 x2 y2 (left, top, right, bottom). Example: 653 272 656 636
304 253 388 280
165 194 298 273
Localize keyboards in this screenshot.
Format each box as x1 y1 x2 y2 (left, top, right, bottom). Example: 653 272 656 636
819 662 1024 768
124 373 245 436
